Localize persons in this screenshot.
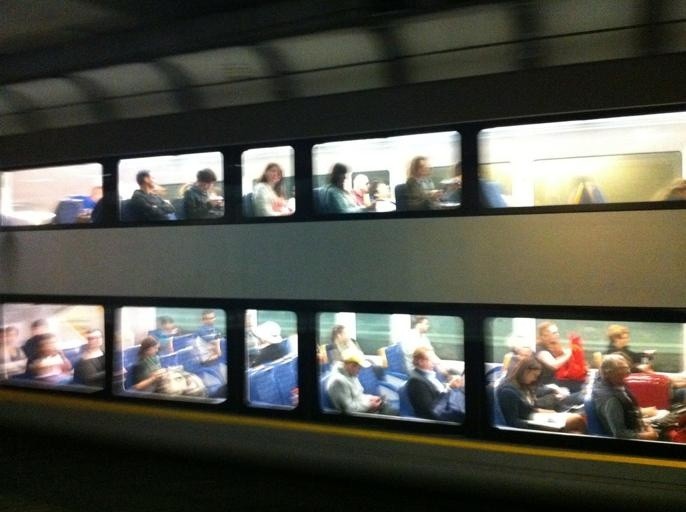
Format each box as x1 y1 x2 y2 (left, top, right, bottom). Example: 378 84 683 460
495 321 685 441
129 310 267 398
317 316 465 420
76 168 225 222
251 162 296 218
2 316 106 388
319 157 506 215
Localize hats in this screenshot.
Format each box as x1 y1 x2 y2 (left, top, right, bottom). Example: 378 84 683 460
250 320 283 344
342 347 372 367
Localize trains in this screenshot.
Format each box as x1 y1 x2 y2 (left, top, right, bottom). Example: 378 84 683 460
0 0 686 511
